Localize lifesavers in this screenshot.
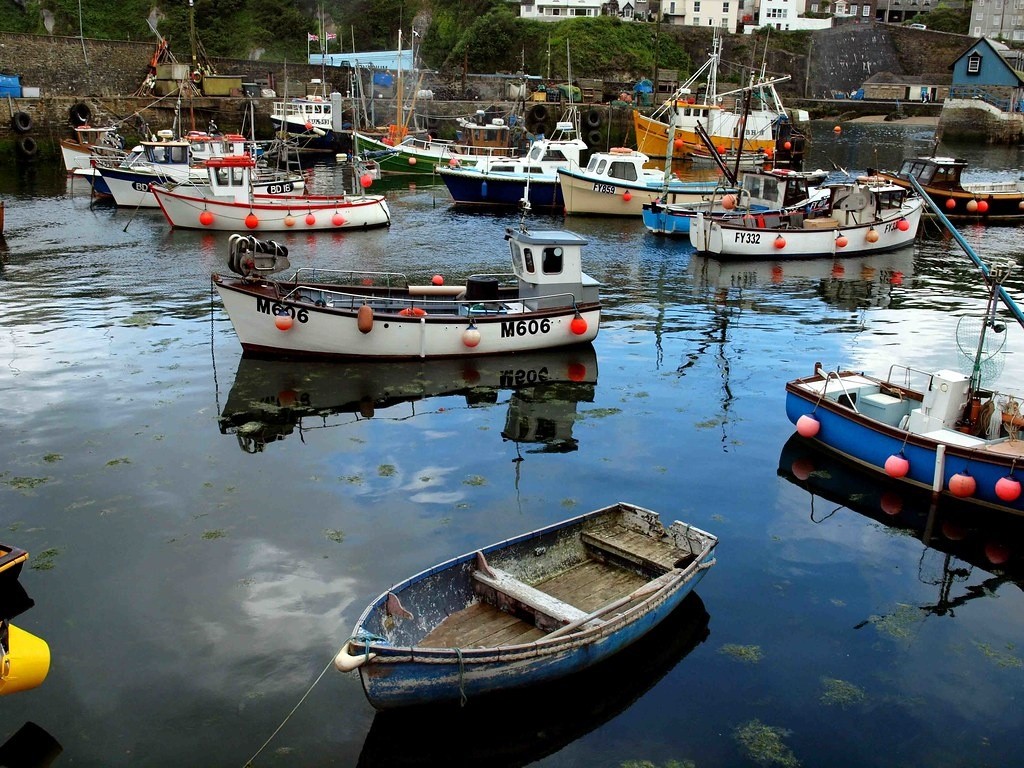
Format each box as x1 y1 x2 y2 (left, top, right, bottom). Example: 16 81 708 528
19 134 38 156
191 71 202 84
69 100 93 122
532 102 549 122
14 110 34 134
587 129 604 145
585 107 602 128
423 110 440 129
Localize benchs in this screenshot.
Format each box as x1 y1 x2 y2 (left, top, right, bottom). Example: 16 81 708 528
582 523 698 581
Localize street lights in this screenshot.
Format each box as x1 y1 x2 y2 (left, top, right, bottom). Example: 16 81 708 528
188 0 197 67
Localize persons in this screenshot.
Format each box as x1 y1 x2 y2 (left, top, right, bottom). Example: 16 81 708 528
919 91 930 103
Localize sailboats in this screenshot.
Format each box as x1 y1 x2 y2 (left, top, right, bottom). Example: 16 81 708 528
352 29 520 174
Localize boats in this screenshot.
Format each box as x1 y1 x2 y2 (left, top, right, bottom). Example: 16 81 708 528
761 168 829 187
691 140 769 165
642 109 831 236
776 429 1024 617
270 94 429 150
212 139 604 361
148 154 391 232
690 168 926 259
94 130 306 210
867 141 1024 220
335 502 720 710
434 105 682 209
60 125 279 196
557 70 742 219
219 342 598 492
633 27 805 170
785 361 1023 516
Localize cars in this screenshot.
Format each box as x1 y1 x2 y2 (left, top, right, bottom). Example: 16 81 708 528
911 24 926 29
